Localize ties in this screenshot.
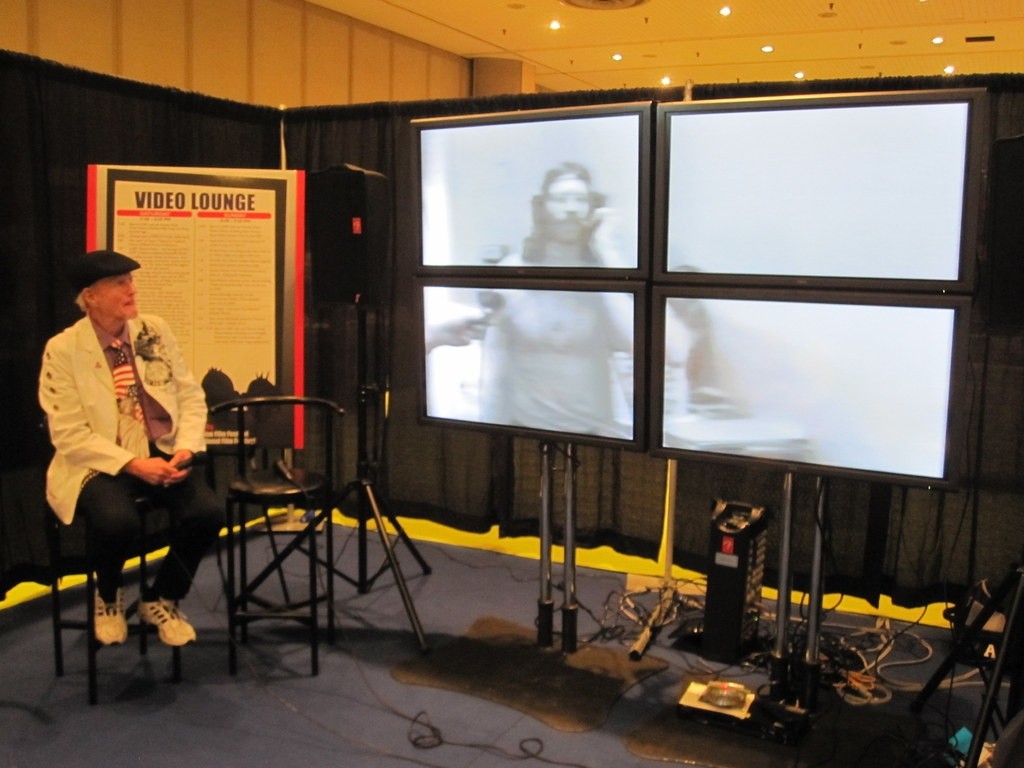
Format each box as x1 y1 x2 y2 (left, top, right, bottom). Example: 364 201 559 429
111 339 145 437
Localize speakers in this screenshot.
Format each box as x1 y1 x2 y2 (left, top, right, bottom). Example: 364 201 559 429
310 164 387 305
985 134 1024 328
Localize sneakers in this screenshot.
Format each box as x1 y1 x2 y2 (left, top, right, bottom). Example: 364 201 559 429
94 584 127 645
137 593 196 646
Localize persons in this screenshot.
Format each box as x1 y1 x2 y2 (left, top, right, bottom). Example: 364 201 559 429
36 250 223 645
425 162 681 439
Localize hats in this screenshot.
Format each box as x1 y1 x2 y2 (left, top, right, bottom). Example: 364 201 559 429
71 250 140 290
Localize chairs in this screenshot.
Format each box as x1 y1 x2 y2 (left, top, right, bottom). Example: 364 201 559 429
209 396 344 677
38 421 185 707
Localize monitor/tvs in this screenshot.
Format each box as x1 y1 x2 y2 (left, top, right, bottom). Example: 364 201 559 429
405 88 989 498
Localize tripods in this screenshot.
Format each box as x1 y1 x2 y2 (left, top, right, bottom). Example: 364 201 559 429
909 562 1024 768
229 302 434 657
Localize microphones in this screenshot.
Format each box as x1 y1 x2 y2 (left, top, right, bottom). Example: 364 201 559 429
175 450 209 472
